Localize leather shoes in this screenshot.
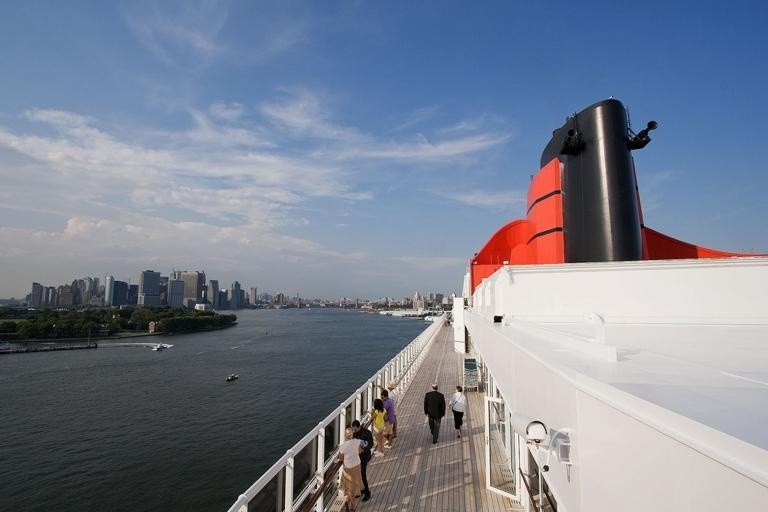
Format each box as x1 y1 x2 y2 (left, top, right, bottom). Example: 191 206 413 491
431 430 437 444
354 492 371 501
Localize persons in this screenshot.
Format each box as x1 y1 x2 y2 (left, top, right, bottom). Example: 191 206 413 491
445 312 451 325
339 420 373 512
424 384 445 444
370 385 394 457
448 386 467 438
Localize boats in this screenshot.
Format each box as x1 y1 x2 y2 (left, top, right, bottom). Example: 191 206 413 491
223 373 239 382
156 342 166 350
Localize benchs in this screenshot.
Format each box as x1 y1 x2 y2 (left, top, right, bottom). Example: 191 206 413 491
325 441 378 512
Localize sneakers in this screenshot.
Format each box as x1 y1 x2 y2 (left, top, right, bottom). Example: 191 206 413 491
374 451 384 457
384 440 392 449
